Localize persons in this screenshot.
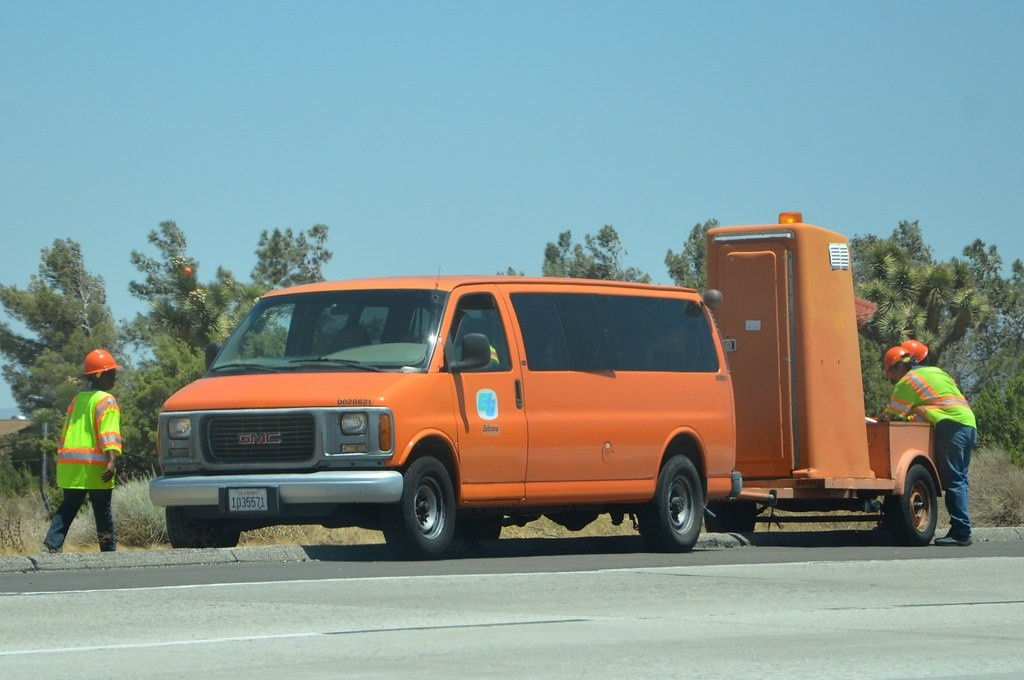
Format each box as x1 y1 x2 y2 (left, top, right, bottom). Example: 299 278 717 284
448 311 500 372
877 346 976 546
900 339 929 367
40 349 122 554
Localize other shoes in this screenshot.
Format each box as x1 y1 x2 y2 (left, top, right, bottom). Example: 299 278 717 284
934 531 972 546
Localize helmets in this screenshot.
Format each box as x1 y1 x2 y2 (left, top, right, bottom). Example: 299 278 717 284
900 340 929 363
884 346 910 378
82 349 121 375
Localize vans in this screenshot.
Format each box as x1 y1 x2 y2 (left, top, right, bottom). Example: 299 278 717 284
150 275 743 561
